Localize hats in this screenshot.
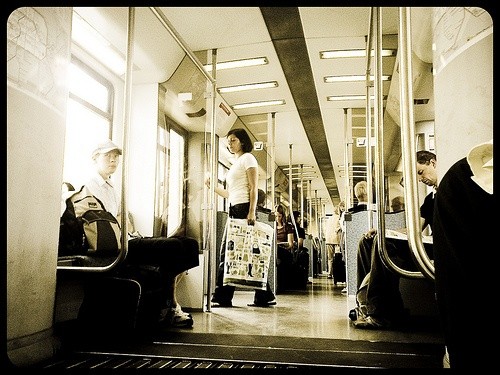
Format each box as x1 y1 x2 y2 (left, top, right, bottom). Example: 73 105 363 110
91 139 123 155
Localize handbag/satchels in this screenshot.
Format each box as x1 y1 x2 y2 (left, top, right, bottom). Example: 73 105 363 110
222 219 273 290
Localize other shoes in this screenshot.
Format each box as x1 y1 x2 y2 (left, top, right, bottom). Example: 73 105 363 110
353 317 384 330
349 306 367 320
175 307 193 328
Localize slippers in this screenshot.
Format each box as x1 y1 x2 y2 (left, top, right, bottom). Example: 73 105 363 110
247 299 277 306
211 302 232 307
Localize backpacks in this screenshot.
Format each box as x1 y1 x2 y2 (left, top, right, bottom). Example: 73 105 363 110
57 182 120 259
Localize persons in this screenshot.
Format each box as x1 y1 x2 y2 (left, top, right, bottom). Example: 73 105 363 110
274 204 309 292
255 189 271 215
391 196 405 212
349 150 438 330
205 128 277 308
73 139 198 329
340 181 376 293
309 229 342 279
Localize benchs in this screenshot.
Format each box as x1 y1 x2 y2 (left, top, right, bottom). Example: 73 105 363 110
321 237 327 274
314 236 323 276
301 234 313 284
345 207 407 298
215 209 278 296
56 254 117 267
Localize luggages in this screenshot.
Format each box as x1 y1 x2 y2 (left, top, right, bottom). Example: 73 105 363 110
332 252 346 284
